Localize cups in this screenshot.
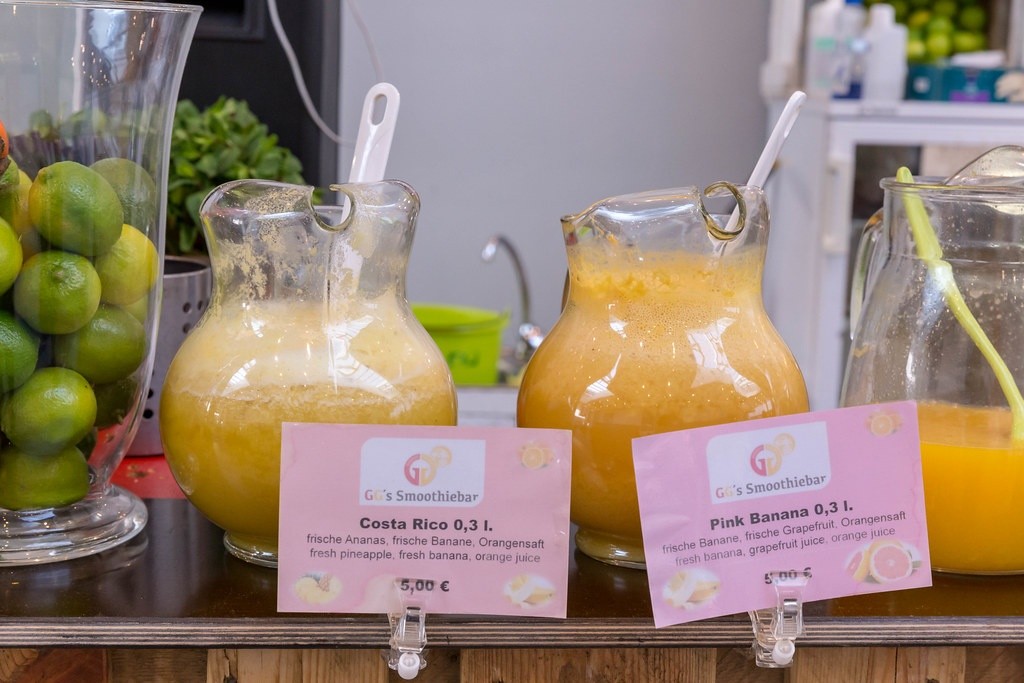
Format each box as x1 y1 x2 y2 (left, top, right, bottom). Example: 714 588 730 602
407 299 509 387
0 0 207 569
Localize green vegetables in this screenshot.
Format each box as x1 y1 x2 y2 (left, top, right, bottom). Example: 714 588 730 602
26 96 318 255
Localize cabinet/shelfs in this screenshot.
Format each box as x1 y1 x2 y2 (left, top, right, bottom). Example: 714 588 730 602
761 101 1024 412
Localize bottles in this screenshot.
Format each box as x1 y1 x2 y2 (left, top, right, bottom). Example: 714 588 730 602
830 144 1024 580
802 1 854 95
158 178 459 570
519 180 810 571
859 4 908 101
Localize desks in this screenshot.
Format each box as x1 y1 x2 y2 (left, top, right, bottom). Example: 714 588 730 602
0 459 1024 683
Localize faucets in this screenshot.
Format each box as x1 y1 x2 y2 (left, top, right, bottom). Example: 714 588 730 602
481 233 538 369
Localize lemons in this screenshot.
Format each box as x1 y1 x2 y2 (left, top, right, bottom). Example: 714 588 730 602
0 155 159 511
